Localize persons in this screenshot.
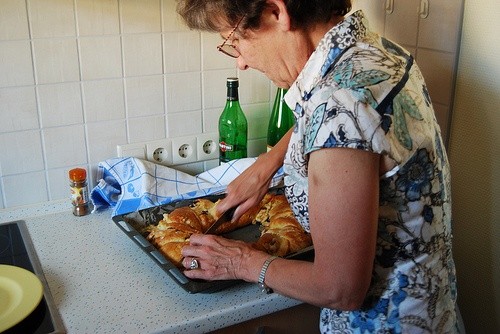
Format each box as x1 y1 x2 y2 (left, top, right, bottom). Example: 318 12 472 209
174 0 459 334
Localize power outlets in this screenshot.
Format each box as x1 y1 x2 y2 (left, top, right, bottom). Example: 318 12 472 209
196 133 220 163
172 136 197 166
147 139 172 168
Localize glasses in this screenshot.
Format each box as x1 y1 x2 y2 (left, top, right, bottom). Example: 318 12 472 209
217 11 244 58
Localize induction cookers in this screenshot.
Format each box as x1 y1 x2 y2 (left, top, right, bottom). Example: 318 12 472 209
0 219 66 334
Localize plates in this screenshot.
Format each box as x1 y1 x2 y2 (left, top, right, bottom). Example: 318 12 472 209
0 264 44 333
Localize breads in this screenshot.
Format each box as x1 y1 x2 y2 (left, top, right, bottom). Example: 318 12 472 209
146 193 313 264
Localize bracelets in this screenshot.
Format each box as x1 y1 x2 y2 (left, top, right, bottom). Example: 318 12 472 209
258 255 279 292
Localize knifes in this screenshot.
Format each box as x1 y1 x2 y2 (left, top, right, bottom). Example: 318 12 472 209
203 208 236 236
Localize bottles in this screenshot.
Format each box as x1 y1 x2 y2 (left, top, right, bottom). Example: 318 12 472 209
265 88 297 152
70 167 91 216
217 78 248 166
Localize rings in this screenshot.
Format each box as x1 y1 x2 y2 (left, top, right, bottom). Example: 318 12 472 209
191 257 198 269
